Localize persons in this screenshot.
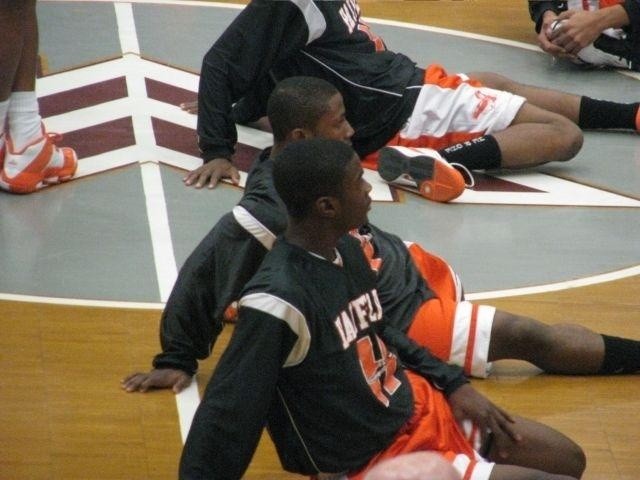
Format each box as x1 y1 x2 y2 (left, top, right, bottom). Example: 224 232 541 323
0 0 77 193
527 0 639 70
175 139 590 480
179 0 640 203
119 78 639 395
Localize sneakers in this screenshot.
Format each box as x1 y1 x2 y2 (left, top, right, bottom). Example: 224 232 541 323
550 18 633 71
377 145 466 203
1 119 79 195
